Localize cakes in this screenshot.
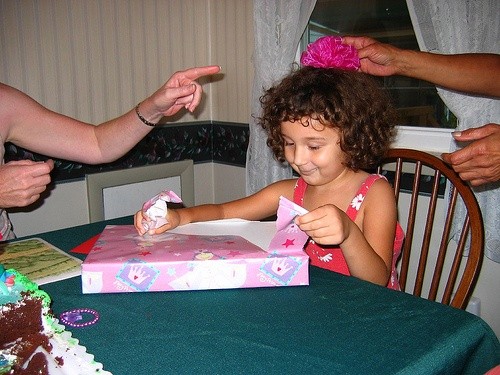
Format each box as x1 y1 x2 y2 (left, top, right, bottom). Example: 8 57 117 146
0 264 64 375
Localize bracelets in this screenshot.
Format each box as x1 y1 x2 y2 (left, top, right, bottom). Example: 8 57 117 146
135 103 159 127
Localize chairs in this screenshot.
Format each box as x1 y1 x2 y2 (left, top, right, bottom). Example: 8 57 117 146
366 140 486 310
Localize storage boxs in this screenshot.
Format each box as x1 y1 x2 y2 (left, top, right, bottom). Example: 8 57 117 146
82 196 316 295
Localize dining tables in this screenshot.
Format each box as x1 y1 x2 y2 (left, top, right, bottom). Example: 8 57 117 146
0 213 500 375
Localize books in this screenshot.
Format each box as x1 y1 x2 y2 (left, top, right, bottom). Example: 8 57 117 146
0 238 84 287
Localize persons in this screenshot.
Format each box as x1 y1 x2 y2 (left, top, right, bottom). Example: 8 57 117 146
0 66 221 242
133 67 405 291
342 37 500 186
